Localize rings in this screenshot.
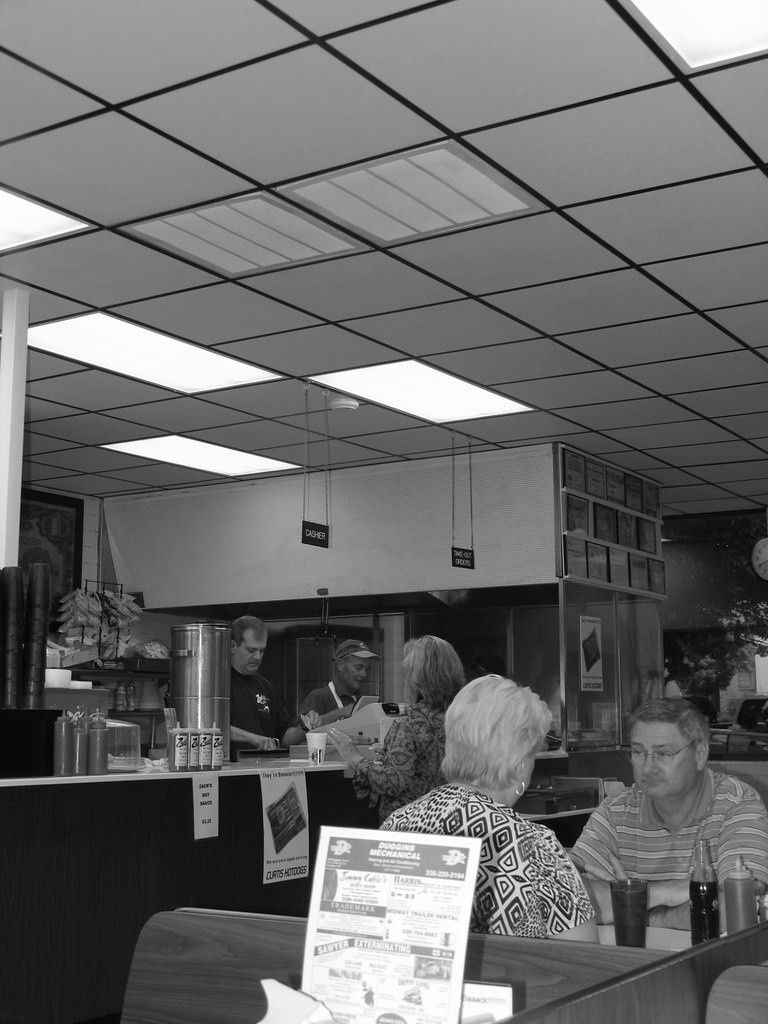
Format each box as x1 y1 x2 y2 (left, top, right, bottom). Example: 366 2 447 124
339 739 341 742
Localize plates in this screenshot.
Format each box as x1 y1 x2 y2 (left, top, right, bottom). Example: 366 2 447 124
104 681 161 710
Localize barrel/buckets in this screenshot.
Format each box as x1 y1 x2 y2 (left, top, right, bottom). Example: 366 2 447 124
168 624 232 764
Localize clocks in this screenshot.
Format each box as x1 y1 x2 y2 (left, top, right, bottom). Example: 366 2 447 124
750 534 768 582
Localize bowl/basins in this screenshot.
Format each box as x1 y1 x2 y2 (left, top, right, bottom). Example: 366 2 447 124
45 669 71 688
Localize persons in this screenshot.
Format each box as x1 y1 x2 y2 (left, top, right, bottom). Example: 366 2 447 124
328 635 467 821
379 674 599 941
229 616 323 763
569 697 768 934
299 639 380 726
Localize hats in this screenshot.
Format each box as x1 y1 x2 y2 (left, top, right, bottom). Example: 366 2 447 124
333 639 382 665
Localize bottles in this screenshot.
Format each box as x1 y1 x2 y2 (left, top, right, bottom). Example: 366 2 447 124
54 707 108 777
116 680 127 712
126 683 136 711
724 855 757 935
689 840 719 948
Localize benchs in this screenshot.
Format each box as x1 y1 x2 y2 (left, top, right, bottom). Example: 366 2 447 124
120 758 768 1024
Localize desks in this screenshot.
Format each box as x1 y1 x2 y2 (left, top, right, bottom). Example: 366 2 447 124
595 924 694 953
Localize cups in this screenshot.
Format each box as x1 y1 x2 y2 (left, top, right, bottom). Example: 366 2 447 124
306 733 327 764
0 562 50 709
609 879 648 948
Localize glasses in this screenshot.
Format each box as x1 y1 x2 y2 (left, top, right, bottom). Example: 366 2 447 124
626 739 701 763
